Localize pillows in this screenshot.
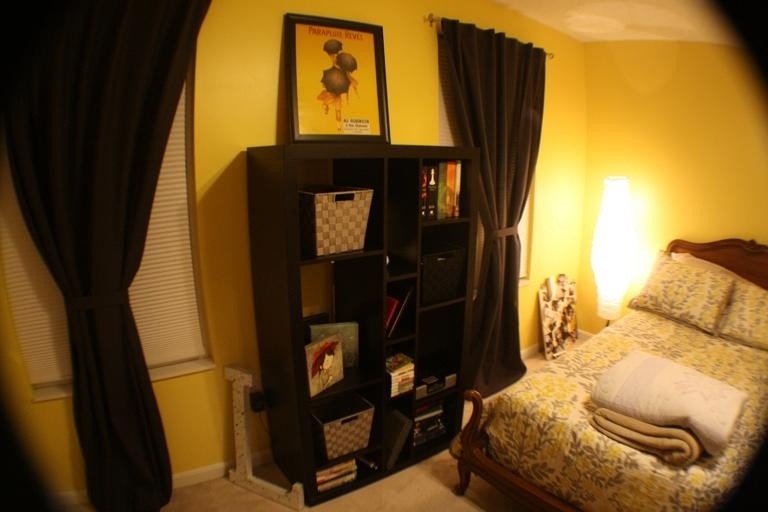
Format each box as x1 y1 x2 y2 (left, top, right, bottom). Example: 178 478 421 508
629 251 737 335
722 277 768 351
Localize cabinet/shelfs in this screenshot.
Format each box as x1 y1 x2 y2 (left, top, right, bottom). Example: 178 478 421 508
247 144 481 508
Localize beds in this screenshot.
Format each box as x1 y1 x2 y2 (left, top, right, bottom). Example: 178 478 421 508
449 239 768 512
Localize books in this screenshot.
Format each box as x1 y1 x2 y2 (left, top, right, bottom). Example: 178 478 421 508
308 322 361 368
412 400 447 446
419 159 463 222
385 288 414 339
313 456 358 493
383 295 400 330
304 331 344 397
385 352 416 399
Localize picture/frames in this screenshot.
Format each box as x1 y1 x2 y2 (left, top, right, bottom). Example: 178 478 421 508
481 162 536 280
285 13 391 145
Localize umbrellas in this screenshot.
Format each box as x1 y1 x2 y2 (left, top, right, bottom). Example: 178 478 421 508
311 341 339 379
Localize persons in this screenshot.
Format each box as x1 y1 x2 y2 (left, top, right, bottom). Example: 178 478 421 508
318 348 335 391
